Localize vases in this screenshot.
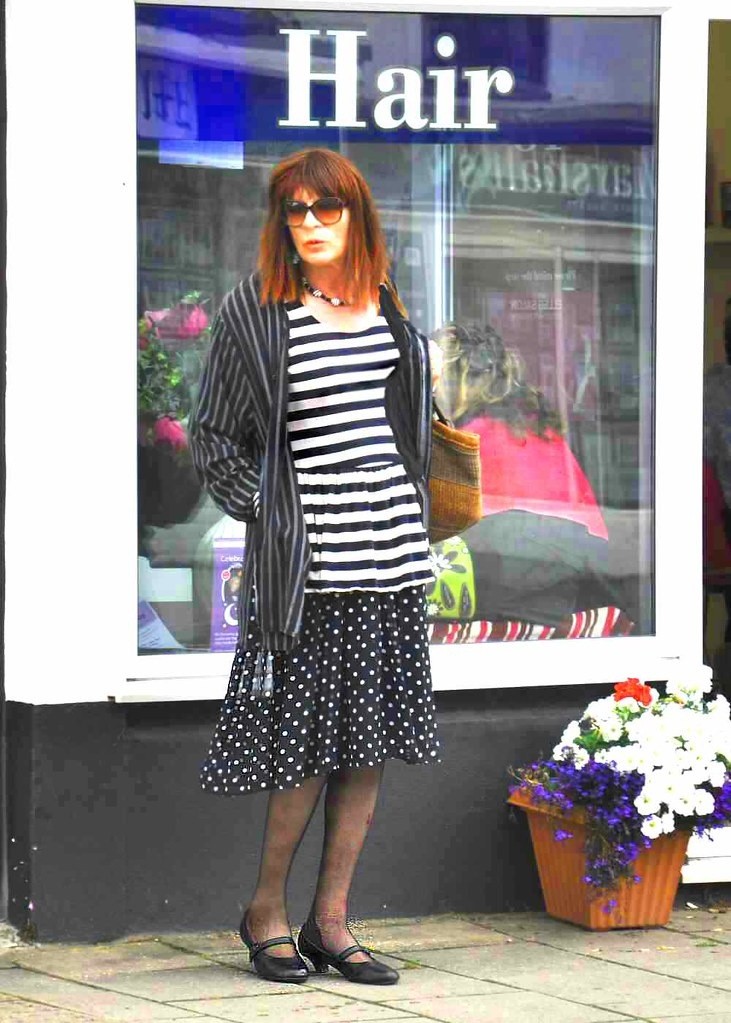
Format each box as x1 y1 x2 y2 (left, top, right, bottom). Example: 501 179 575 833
506 787 688 932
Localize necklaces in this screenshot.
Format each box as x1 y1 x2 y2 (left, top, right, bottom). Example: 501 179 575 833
293 255 361 306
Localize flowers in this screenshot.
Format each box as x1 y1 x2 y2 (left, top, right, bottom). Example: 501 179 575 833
140 288 213 451
498 661 731 918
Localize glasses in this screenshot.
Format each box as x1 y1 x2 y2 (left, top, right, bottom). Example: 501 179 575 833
284 197 349 227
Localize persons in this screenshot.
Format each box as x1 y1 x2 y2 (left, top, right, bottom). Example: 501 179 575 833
188 149 432 982
431 323 627 645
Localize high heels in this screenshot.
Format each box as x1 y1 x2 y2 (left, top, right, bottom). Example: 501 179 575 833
297 923 399 985
240 909 308 983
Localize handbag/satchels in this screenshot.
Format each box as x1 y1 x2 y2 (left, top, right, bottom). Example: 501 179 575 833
426 399 481 545
